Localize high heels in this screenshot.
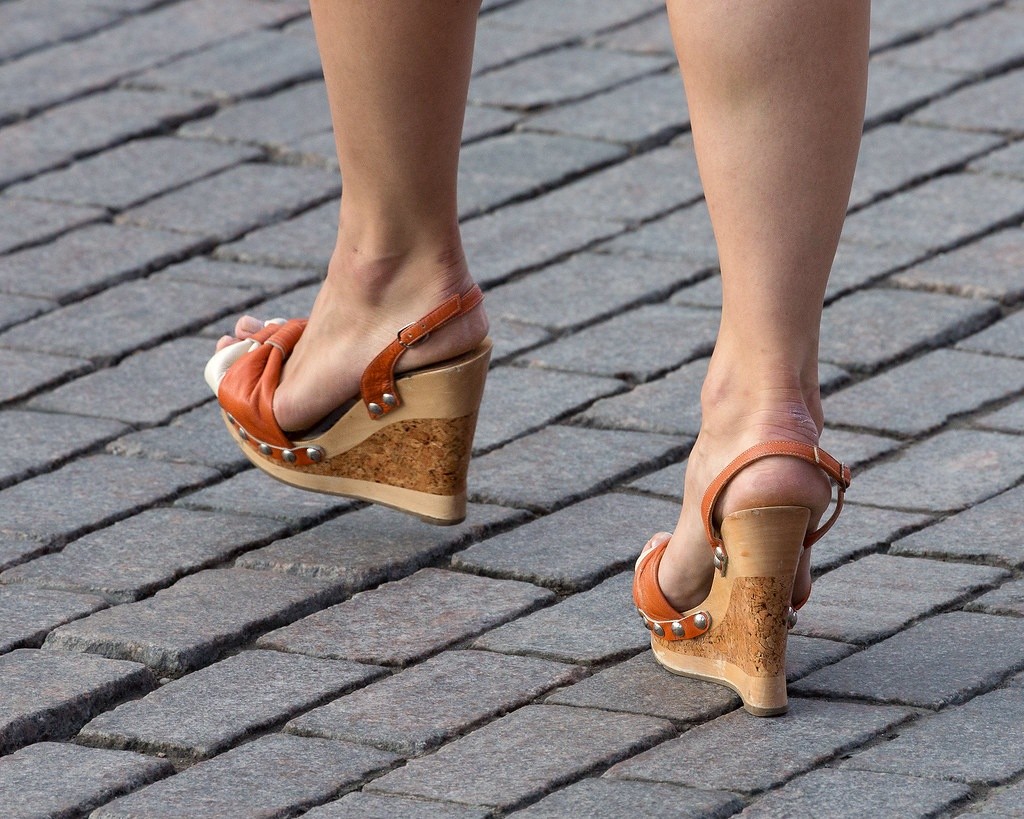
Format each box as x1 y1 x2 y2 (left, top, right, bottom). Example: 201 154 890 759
203 283 493 526
633 438 852 717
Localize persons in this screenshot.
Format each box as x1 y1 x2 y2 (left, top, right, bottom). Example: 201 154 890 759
204 0 870 721
